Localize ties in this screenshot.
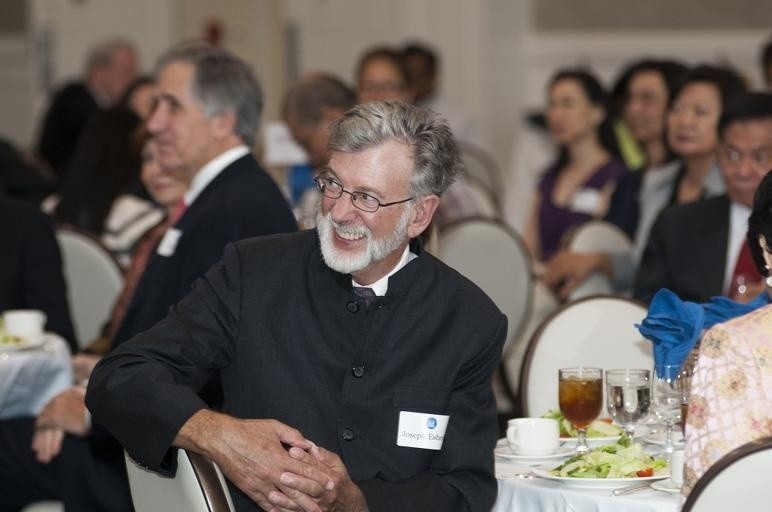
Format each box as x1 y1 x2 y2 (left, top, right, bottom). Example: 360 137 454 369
173 198 187 224
728 239 761 300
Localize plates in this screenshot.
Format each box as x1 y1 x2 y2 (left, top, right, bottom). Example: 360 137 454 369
1 337 48 353
494 448 581 463
558 424 650 445
529 466 671 491
651 478 684 500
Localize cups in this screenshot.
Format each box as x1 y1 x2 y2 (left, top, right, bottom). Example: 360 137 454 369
506 418 562 455
3 308 47 342
667 449 686 492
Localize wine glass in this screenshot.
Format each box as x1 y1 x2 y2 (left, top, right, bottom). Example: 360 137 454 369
652 363 683 460
558 367 604 454
605 366 651 452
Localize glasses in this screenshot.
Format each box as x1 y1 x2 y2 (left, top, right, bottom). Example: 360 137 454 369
313 171 413 213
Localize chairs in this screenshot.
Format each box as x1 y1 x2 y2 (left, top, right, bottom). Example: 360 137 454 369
679 438 772 511
57 227 130 350
121 444 237 511
422 139 680 418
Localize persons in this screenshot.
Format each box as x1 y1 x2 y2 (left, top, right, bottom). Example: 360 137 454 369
1 38 298 511
636 91 772 308
84 100 510 512
683 303 771 496
282 43 440 252
525 59 749 300
640 171 771 379
